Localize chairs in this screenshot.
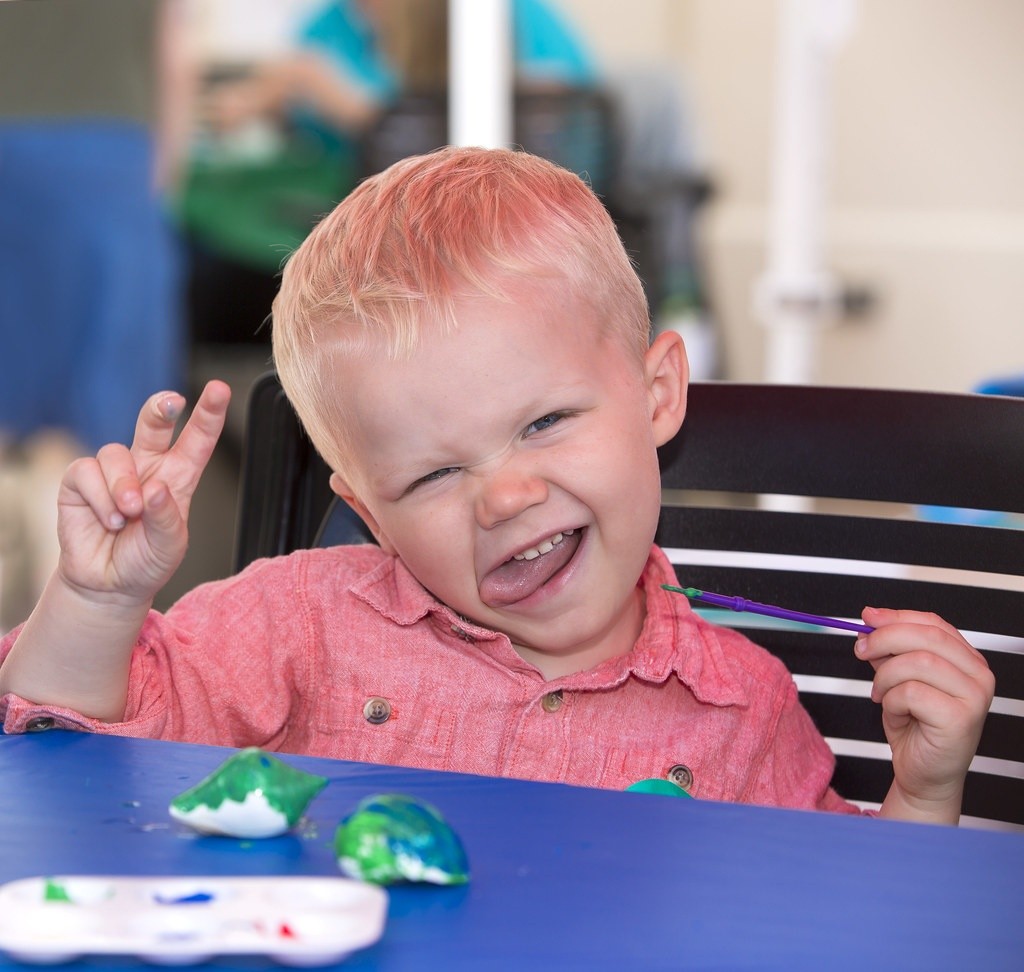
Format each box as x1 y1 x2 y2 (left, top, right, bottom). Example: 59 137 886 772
231 375 1024 837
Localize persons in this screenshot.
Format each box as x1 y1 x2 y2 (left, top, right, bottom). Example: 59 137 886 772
0 143 998 828
180 0 605 165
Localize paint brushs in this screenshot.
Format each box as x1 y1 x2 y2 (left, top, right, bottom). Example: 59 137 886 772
659 582 875 634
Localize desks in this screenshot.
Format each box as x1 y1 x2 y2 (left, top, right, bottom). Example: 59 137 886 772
0 731 1023 971
